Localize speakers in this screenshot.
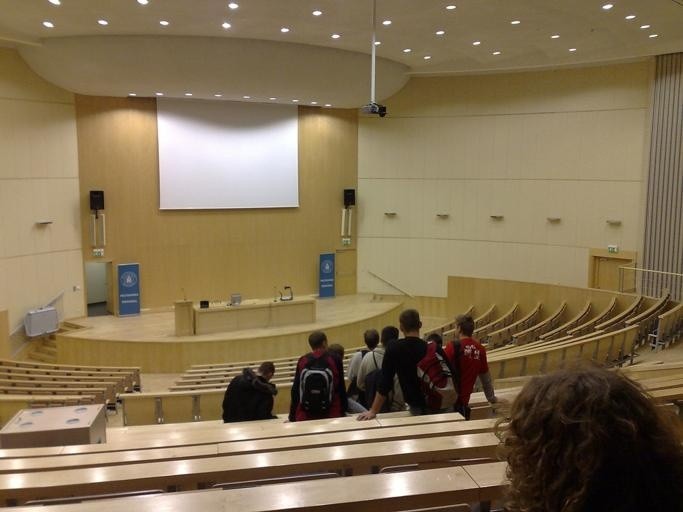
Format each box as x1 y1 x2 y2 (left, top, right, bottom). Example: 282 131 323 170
90 190 104 210
344 188 356 207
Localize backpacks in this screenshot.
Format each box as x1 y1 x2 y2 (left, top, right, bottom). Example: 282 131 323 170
366 351 396 413
223 373 256 418
299 353 334 414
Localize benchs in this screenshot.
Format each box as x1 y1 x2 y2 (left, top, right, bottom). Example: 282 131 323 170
344 289 683 357
1 358 682 511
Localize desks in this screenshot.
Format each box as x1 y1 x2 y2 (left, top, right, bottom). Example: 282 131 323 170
175 293 317 334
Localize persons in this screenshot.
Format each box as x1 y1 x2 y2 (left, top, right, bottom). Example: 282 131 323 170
283 309 508 425
492 363 683 511
222 361 279 423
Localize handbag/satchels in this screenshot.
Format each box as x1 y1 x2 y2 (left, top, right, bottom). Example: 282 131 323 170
416 352 458 410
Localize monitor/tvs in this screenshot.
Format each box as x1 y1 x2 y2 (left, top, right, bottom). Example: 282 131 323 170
231 293 242 305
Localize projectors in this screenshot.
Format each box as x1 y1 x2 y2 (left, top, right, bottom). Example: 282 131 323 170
360 102 387 119
